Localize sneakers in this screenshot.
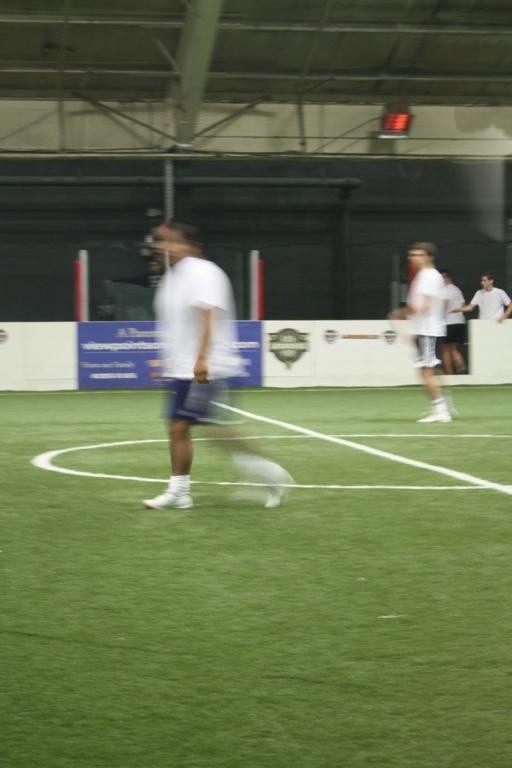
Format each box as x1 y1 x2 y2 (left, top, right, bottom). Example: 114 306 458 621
418 416 451 423
263 474 289 507
141 492 192 511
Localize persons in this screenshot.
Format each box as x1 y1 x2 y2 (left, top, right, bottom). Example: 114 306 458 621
446 273 512 324
102 251 174 290
437 270 466 375
384 242 459 425
136 219 297 511
